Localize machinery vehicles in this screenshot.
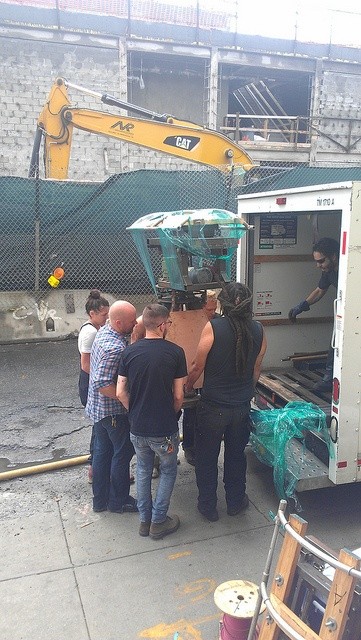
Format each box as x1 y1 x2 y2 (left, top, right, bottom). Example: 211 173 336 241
26 76 249 177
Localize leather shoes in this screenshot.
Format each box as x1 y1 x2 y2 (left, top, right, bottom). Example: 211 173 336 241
149 514 180 541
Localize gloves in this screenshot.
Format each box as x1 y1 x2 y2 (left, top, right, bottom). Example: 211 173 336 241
289 300 310 323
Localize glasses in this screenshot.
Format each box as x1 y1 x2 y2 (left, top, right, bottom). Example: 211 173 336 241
314 257 326 263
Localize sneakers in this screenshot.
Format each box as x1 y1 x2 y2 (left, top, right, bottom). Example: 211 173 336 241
314 379 332 392
226 494 248 516
197 503 218 521
108 495 140 512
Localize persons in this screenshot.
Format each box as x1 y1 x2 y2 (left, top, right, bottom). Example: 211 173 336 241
77 289 110 483
288 238 340 392
185 287 222 466
84 300 140 513
115 304 188 541
184 283 266 522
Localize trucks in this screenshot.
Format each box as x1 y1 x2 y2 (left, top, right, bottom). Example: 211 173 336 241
236 177 361 491
